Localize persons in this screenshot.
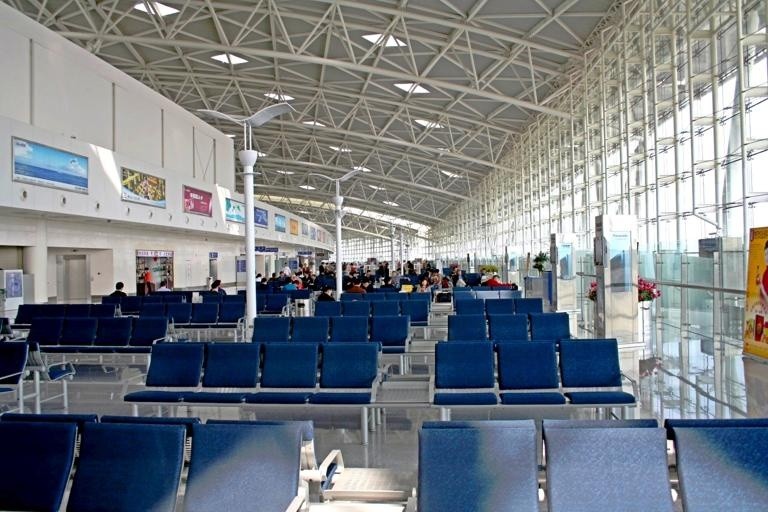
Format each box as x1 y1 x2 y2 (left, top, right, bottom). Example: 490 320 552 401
211 283 220 296
215 280 226 294
143 268 153 294
256 258 514 300
157 281 171 291
111 283 126 296
